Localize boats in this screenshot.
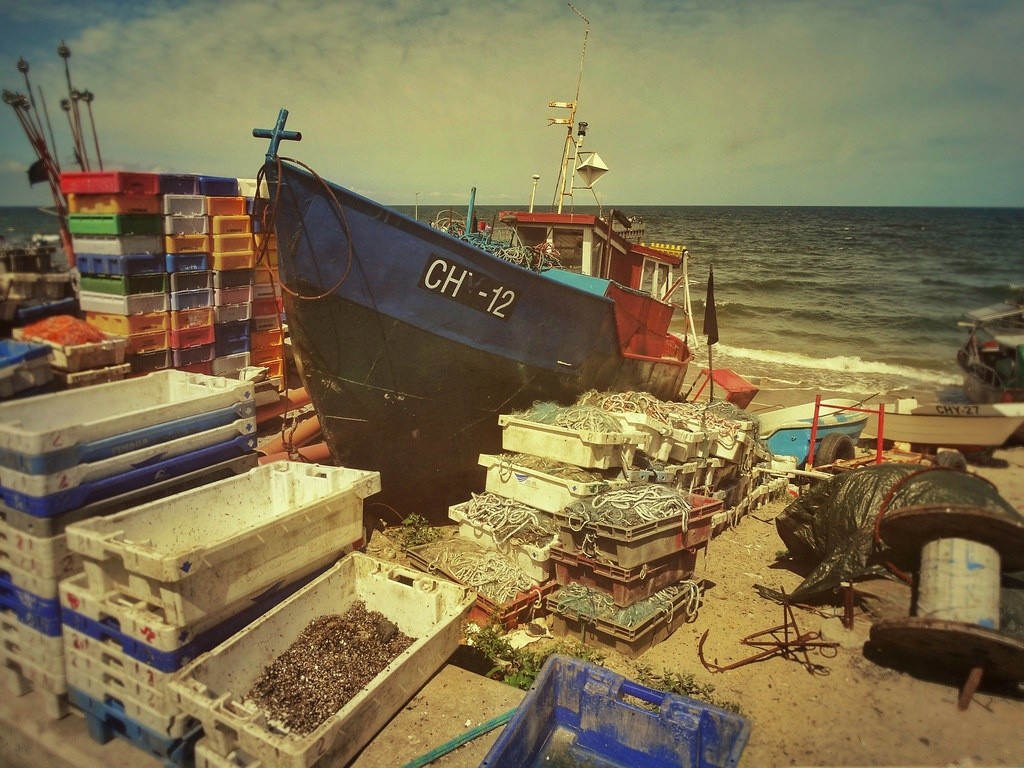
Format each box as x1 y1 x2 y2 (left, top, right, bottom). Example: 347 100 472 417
860 402 1024 464
956 300 1024 406
253 0 699 525
752 398 872 470
0 234 79 330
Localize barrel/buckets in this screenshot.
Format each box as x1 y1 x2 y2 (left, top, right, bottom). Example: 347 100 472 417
915 537 1001 632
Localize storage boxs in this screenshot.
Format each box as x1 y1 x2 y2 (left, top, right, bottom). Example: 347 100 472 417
0 170 798 768
